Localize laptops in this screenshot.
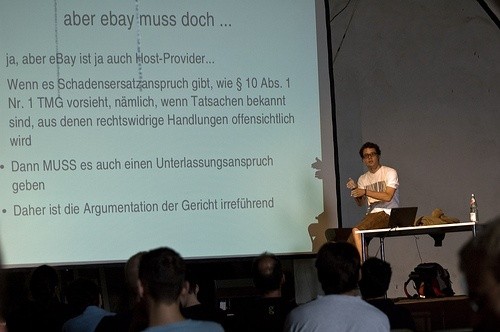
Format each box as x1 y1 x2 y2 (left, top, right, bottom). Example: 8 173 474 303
386 206 418 228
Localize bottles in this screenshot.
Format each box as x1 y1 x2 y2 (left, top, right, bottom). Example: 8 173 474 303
470 194 479 222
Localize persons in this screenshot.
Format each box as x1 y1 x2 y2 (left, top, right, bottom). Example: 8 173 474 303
0 247 228 332
346 142 399 267
236 253 298 332
284 242 391 332
459 215 500 332
359 257 415 332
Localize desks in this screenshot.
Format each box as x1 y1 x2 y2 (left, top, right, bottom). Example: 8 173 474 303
355 222 478 301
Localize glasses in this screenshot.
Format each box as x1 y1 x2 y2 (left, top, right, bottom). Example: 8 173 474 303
363 152 377 159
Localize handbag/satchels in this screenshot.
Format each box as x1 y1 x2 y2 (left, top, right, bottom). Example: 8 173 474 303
412 263 454 296
